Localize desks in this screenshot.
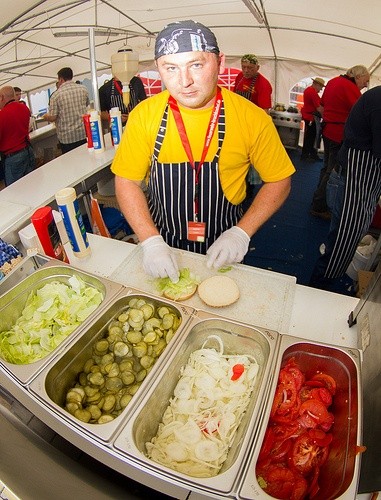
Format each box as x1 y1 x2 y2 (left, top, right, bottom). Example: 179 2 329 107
28 123 58 145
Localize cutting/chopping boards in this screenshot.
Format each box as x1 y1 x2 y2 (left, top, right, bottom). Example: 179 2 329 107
109 244 296 334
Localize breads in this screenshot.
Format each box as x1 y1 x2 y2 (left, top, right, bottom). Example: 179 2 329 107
163 283 198 302
197 275 240 308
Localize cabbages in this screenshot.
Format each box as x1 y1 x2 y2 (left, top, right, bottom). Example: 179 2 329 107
149 267 197 298
0 275 89 365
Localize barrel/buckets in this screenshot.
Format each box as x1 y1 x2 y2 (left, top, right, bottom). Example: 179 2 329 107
345 248 371 281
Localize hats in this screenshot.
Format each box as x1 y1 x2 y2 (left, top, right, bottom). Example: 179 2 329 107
311 77 326 87
154 20 220 60
241 54 259 65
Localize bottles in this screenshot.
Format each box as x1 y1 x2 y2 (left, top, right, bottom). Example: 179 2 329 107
30 207 71 264
109 106 122 147
89 110 105 151
54 187 92 257
83 115 94 148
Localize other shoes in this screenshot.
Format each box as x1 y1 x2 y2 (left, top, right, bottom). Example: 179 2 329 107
310 208 331 219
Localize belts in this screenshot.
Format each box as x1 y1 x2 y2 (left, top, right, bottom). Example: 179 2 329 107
5 146 28 159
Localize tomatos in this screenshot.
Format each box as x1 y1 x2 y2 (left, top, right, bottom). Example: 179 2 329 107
256 355 337 500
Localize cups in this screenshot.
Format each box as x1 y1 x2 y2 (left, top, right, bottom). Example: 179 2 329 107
52 210 70 245
19 223 46 255
104 132 113 147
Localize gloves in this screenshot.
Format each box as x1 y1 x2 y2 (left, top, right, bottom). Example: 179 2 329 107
206 226 250 270
141 235 180 284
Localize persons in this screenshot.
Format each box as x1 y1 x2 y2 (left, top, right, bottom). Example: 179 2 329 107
232 55 273 113
110 20 297 284
310 85 381 295
0 67 146 187
311 65 369 219
302 78 326 161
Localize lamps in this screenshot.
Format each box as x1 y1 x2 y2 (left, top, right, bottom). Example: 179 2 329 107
243 0 264 24
53 31 118 37
111 44 138 107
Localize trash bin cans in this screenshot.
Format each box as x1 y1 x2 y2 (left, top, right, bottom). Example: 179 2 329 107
346 248 381 282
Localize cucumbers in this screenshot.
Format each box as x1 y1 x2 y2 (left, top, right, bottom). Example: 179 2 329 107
63 298 181 424
